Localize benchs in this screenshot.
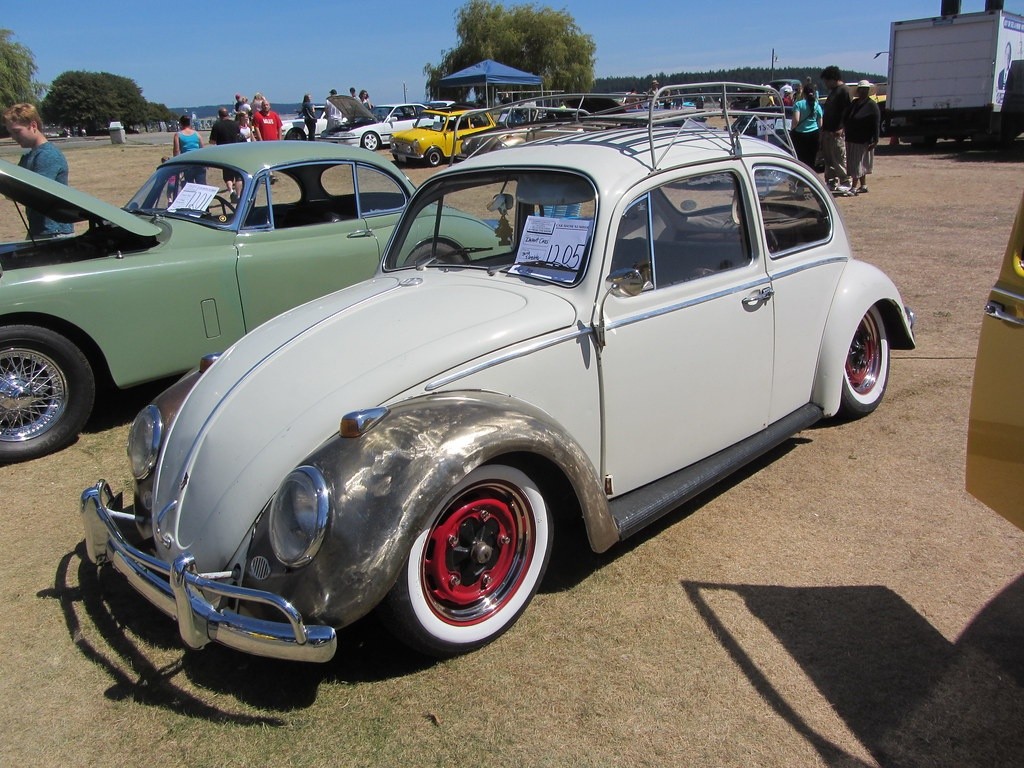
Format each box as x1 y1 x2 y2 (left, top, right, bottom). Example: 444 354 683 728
280 205 339 228
613 238 751 291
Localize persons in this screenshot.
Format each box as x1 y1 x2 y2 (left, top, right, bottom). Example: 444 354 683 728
664 89 685 110
207 108 243 203
997 42 1016 90
817 63 852 192
172 114 209 186
1 103 76 241
559 102 567 109
787 82 823 196
359 90 372 110
628 88 641 109
692 88 705 109
350 87 363 105
232 92 283 185
747 75 817 108
843 79 881 196
161 156 176 207
646 79 661 109
324 89 344 130
302 93 317 141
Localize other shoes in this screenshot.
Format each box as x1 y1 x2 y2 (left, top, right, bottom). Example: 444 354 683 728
796 187 805 195
836 182 852 193
857 186 869 193
843 188 859 197
230 191 240 204
269 174 277 184
825 181 838 190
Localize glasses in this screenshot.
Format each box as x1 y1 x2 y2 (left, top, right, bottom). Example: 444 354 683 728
793 88 799 91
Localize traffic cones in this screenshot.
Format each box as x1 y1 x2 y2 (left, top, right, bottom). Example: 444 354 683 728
888 134 898 147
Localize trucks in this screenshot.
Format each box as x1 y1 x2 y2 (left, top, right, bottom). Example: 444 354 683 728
884 8 1024 155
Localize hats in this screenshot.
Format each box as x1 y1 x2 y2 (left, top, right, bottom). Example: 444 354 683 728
329 89 337 95
235 94 240 99
856 80 875 88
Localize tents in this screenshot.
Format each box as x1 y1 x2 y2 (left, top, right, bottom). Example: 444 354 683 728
437 58 545 109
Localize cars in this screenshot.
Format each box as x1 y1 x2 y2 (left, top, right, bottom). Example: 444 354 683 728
1 140 511 467
460 92 708 158
280 105 347 140
80 79 916 667
389 108 496 168
852 94 888 138
724 106 824 185
320 95 456 151
